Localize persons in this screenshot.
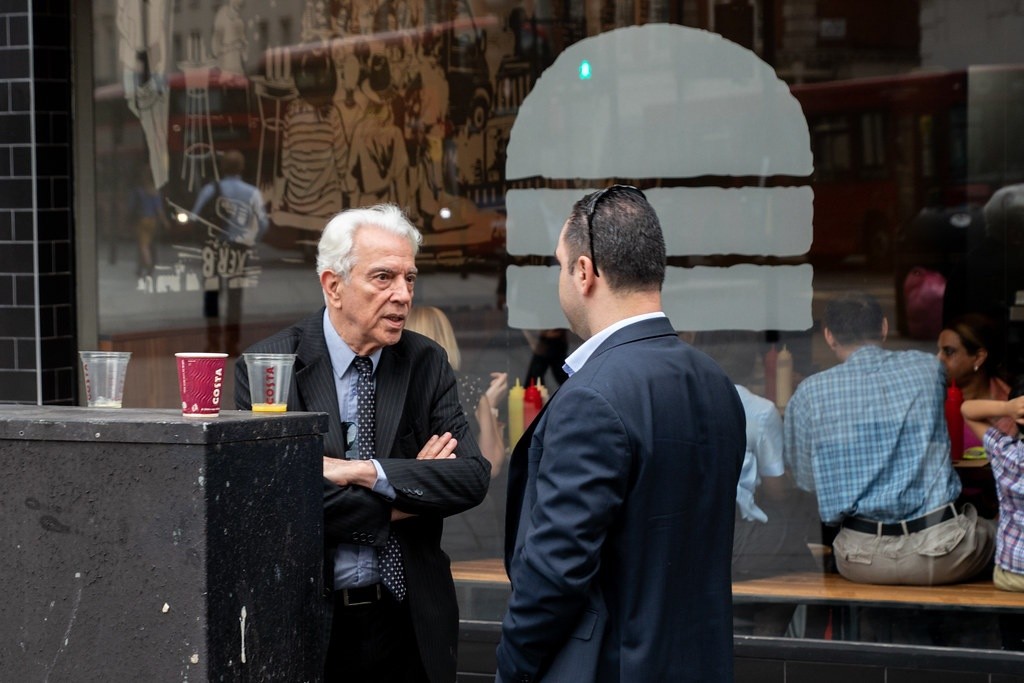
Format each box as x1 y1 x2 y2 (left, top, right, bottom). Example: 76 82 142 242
234 205 492 683
497 249 569 389
189 151 269 358
495 183 747 683
732 185 1024 591
134 173 169 277
403 306 508 478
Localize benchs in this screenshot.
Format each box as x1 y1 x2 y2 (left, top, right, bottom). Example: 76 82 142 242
450 558 1024 683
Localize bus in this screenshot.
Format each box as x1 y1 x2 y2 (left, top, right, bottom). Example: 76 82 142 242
780 66 1024 278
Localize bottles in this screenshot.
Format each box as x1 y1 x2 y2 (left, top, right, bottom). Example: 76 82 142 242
535 378 550 405
764 343 777 401
775 344 793 409
523 378 544 431
507 377 525 449
945 378 965 462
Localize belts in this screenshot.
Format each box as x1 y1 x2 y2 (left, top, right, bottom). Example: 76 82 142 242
840 498 959 535
333 581 390 609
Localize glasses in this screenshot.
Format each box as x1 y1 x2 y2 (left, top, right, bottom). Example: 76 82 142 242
584 186 646 277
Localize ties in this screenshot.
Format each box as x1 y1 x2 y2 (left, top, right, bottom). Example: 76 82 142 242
353 354 407 599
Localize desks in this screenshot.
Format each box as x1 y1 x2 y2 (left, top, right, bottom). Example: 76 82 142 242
951 459 992 475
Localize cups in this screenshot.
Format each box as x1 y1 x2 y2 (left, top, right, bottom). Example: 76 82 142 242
242 353 299 413
175 352 229 418
79 350 132 408
